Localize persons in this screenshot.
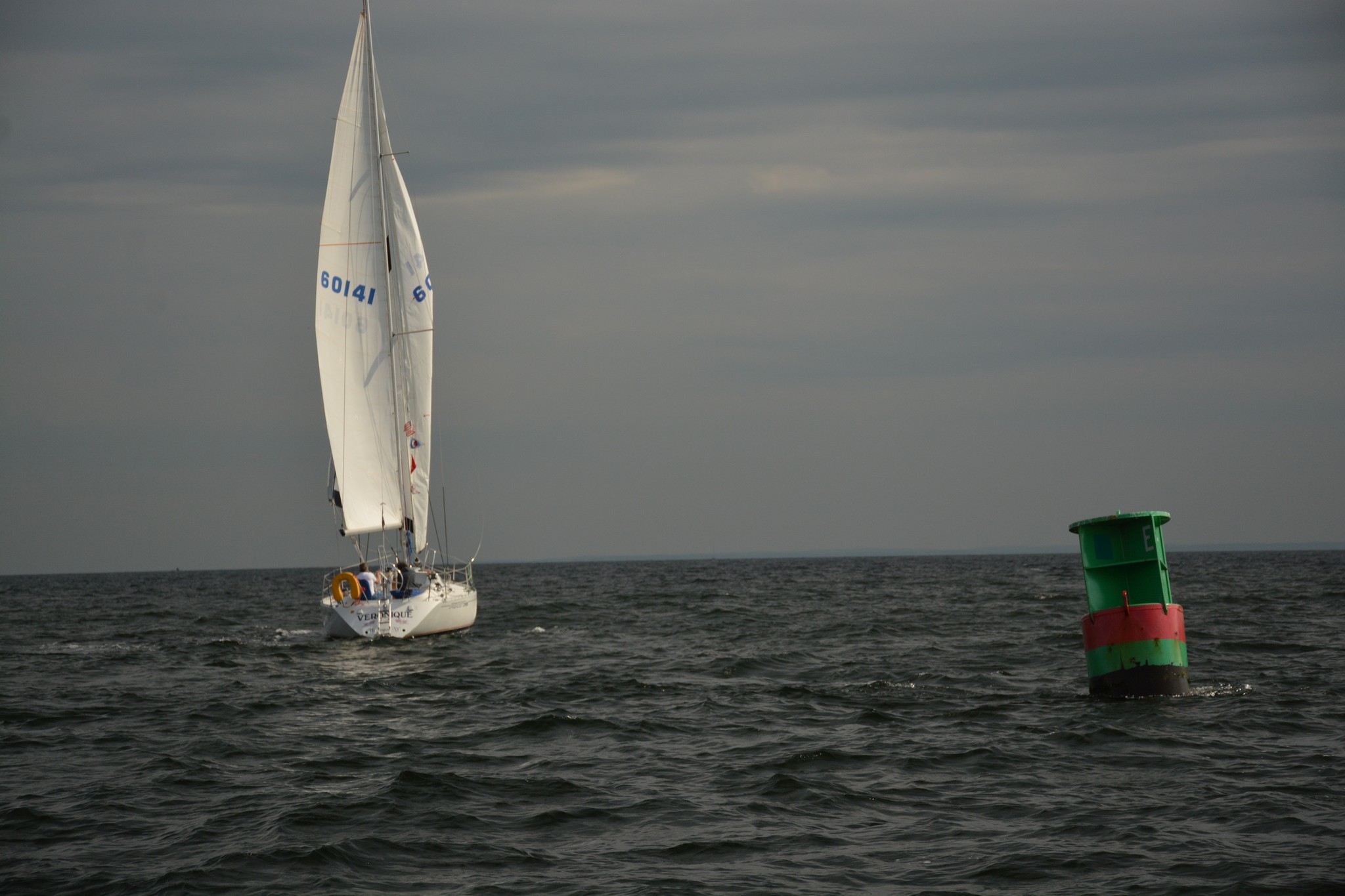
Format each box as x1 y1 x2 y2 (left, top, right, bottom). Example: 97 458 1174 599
354 563 410 601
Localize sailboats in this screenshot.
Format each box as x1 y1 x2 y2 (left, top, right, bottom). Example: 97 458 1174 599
308 1 478 643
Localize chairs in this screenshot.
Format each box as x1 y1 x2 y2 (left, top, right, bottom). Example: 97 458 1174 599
358 580 373 600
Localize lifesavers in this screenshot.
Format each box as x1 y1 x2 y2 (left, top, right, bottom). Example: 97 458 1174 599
332 572 360 601
